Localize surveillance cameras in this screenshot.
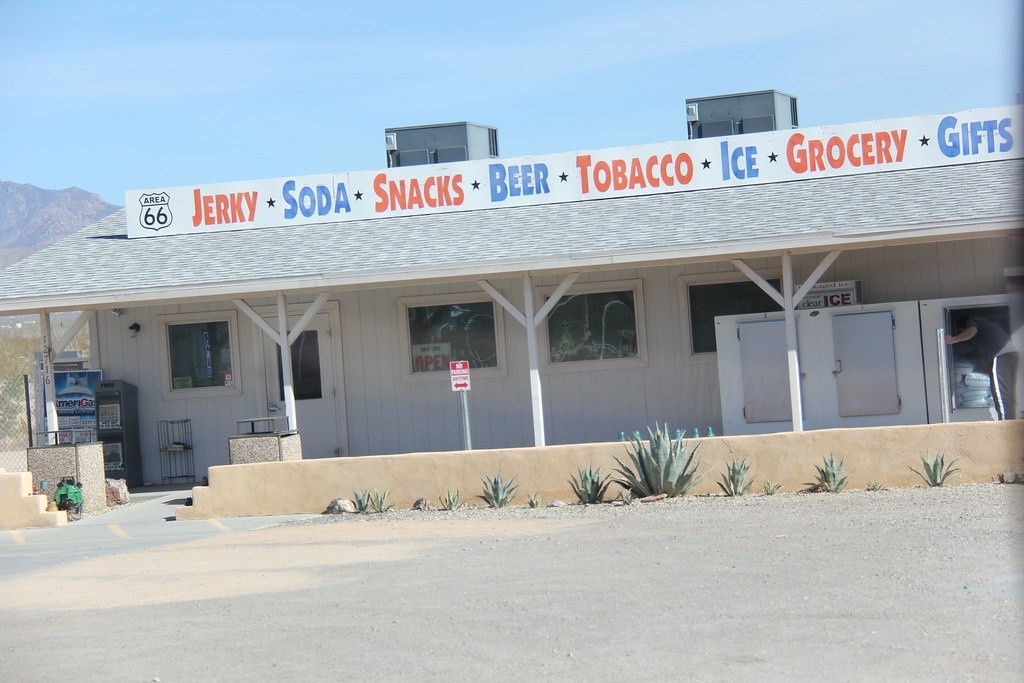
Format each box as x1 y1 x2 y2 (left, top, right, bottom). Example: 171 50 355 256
111 309 121 317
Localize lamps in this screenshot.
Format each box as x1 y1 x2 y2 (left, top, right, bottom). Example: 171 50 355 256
128 323 140 338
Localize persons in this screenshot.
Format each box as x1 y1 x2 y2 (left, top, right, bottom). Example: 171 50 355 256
943 315 1021 422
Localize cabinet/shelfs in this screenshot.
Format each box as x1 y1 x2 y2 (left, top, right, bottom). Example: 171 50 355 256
157 418 195 485
